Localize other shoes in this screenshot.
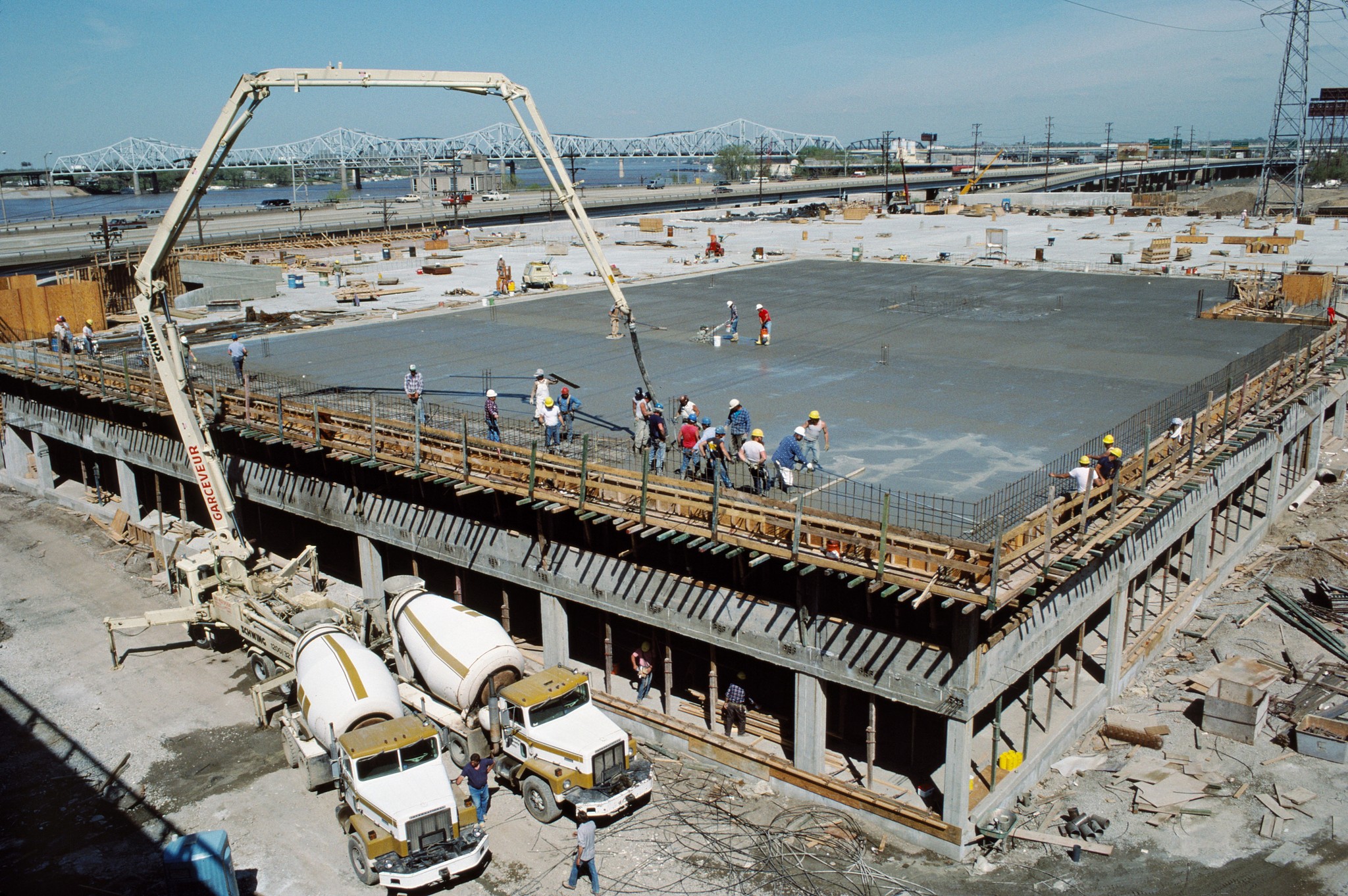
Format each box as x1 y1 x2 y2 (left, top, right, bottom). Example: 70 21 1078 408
590 888 600 896
563 881 575 889
483 815 488 820
637 694 652 704
479 822 485 828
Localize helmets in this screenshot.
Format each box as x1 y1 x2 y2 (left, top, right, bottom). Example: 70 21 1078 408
86 319 93 325
1077 434 1122 465
737 671 746 679
486 389 497 397
634 386 820 437
561 387 569 395
642 642 650 652
727 301 733 307
180 336 188 344
335 260 340 264
535 369 543 375
443 226 446 229
545 397 553 407
232 333 238 339
57 317 62 321
499 254 503 258
756 304 763 310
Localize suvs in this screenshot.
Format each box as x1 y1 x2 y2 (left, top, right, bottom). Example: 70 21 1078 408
255 198 291 210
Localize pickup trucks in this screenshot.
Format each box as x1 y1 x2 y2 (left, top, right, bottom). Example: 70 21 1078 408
481 191 510 202
98 218 148 232
441 195 473 205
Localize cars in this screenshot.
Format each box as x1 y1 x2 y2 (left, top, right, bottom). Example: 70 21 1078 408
882 172 892 176
712 181 731 186
137 209 165 219
912 171 923 175
1058 162 1068 166
778 177 795 182
394 194 421 203
712 186 733 193
1183 159 1192 161
938 168 948 173
807 176 819 180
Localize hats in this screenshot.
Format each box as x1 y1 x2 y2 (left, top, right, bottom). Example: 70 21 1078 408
410 365 416 371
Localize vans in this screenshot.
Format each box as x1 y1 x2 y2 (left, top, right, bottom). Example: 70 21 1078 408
645 178 666 190
851 172 866 178
750 177 769 184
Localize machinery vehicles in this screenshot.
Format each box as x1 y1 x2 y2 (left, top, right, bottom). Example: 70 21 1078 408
887 137 913 215
103 61 657 896
1311 178 1342 189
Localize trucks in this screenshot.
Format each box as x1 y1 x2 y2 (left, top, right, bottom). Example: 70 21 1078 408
1076 155 1095 165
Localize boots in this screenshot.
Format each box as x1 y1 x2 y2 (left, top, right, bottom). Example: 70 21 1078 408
730 332 771 345
240 379 244 385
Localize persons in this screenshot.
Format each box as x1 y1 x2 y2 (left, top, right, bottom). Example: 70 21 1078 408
1238 209 1247 226
180 336 197 369
54 316 97 360
497 255 505 275
456 753 495 828
484 389 501 442
631 641 656 705
1049 435 1122 533
609 304 623 338
632 387 830 497
138 323 148 357
404 364 425 426
228 334 247 385
332 260 344 288
724 671 748 737
462 226 470 242
432 226 448 240
530 368 581 448
756 304 772 345
562 810 600 896
727 301 739 341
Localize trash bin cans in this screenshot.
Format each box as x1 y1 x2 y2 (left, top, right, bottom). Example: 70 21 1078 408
802 230 808 240
852 247 860 261
409 247 416 257
288 274 296 289
295 275 304 288
1035 248 1044 262
383 248 391 260
354 250 361 260
668 227 673 236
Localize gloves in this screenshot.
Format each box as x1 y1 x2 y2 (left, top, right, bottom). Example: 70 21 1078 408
193 357 198 362
806 463 814 471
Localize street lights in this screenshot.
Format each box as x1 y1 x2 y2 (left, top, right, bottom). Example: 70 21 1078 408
0 150 8 227
417 149 423 205
1054 145 1063 175
44 152 55 215
845 144 851 177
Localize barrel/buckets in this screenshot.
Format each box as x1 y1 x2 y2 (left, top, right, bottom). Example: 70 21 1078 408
826 540 840 561
439 301 444 308
288 274 296 288
726 325 731 332
852 248 860 261
760 328 767 337
488 299 494 305
1216 211 1221 219
319 272 329 286
481 299 487 306
668 227 673 237
1186 269 1190 274
382 248 390 259
1192 269 1197 274
353 246 358 250
713 336 721 347
296 275 304 288
755 247 763 256
354 250 361 260
409 247 415 256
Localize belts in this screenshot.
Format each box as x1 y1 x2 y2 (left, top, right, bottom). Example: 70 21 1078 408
804 439 814 442
